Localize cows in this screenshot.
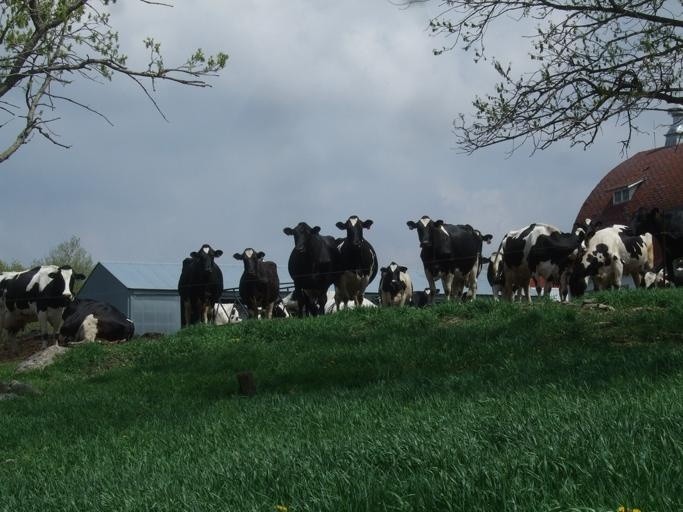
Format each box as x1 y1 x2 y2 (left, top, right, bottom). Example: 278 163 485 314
176 241 240 324
232 246 282 323
406 215 682 305
64 294 135 347
279 215 415 322
0 261 85 347
